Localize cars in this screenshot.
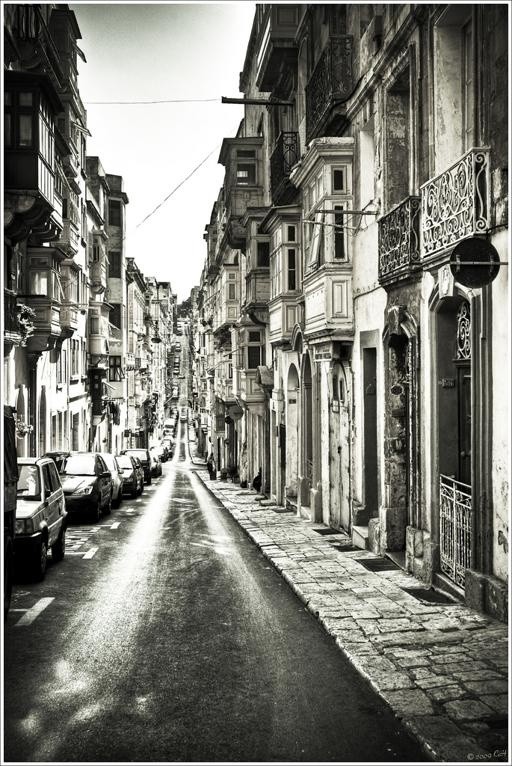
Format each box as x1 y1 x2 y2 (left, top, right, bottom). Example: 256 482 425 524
152 408 179 462
41 448 162 525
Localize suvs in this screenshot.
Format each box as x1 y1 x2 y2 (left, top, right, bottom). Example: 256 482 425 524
13 454 69 586
